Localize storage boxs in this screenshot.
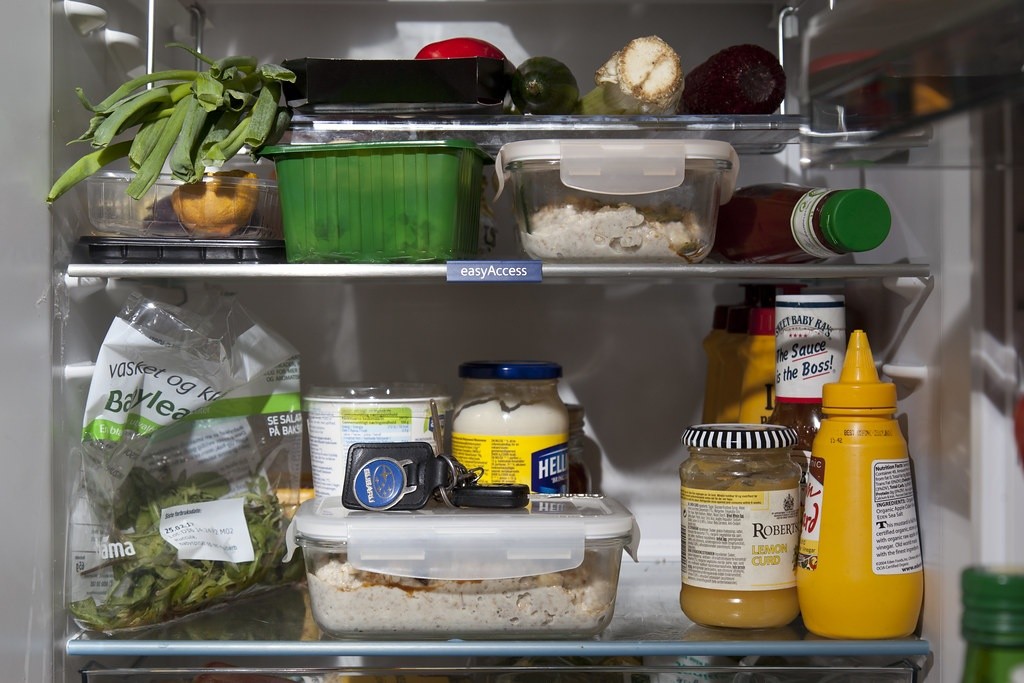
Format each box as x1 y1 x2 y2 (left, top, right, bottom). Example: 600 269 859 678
83 160 274 236
262 136 481 260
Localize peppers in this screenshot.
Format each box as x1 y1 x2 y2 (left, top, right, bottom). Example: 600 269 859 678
507 57 580 115
414 36 508 59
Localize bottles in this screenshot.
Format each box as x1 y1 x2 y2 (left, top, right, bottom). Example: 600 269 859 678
449 359 569 495
702 283 847 499
709 184 892 264
796 329 925 640
564 404 593 496
956 563 1024 683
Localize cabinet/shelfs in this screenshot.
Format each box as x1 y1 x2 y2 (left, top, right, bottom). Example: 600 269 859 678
58 103 949 663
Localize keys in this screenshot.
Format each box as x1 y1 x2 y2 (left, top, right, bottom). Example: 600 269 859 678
429 399 605 508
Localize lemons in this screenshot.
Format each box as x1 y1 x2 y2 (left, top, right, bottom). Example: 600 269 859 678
171 169 261 238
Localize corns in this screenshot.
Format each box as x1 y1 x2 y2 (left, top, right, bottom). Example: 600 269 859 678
676 43 785 114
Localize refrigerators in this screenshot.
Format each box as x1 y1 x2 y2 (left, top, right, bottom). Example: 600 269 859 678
0 0 1024 683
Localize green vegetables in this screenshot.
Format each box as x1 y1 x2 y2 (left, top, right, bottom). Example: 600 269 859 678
70 463 305 635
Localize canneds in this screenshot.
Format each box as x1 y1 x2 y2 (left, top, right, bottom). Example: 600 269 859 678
678 423 802 630
446 359 571 499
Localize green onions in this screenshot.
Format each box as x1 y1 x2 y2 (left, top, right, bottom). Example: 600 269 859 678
46 43 297 204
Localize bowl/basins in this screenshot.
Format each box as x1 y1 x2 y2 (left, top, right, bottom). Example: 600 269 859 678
492 137 741 267
283 489 640 640
